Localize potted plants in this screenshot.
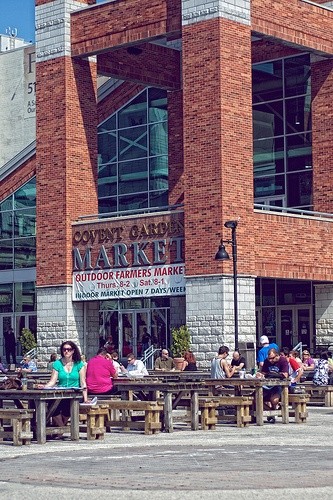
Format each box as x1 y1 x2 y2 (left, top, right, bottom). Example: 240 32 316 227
170 324 192 370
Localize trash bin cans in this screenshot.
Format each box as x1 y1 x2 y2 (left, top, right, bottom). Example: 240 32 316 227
238 341 255 371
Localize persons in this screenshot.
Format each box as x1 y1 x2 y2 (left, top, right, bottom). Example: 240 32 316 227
249 348 289 424
86 347 123 395
119 353 150 376
0 356 9 374
112 352 128 376
81 354 88 370
38 341 91 439
257 334 333 386
4 328 18 364
231 351 246 371
47 353 58 373
181 351 197 371
15 356 37 379
211 346 236 389
155 349 176 371
140 327 151 361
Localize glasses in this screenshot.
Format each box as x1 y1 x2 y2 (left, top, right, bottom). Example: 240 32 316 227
62 348 75 352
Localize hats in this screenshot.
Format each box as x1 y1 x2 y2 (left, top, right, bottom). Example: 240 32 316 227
259 335 269 345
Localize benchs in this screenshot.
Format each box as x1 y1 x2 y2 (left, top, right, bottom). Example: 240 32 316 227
98 401 163 435
288 394 311 424
304 385 333 407
0 409 35 446
199 396 254 431
79 404 109 440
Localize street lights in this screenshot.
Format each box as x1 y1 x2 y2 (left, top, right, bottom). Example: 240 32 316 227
214 221 238 354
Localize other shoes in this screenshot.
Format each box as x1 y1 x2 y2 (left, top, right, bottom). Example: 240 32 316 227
55 435 71 440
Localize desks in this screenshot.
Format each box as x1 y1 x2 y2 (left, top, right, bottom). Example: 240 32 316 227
0 359 333 446
